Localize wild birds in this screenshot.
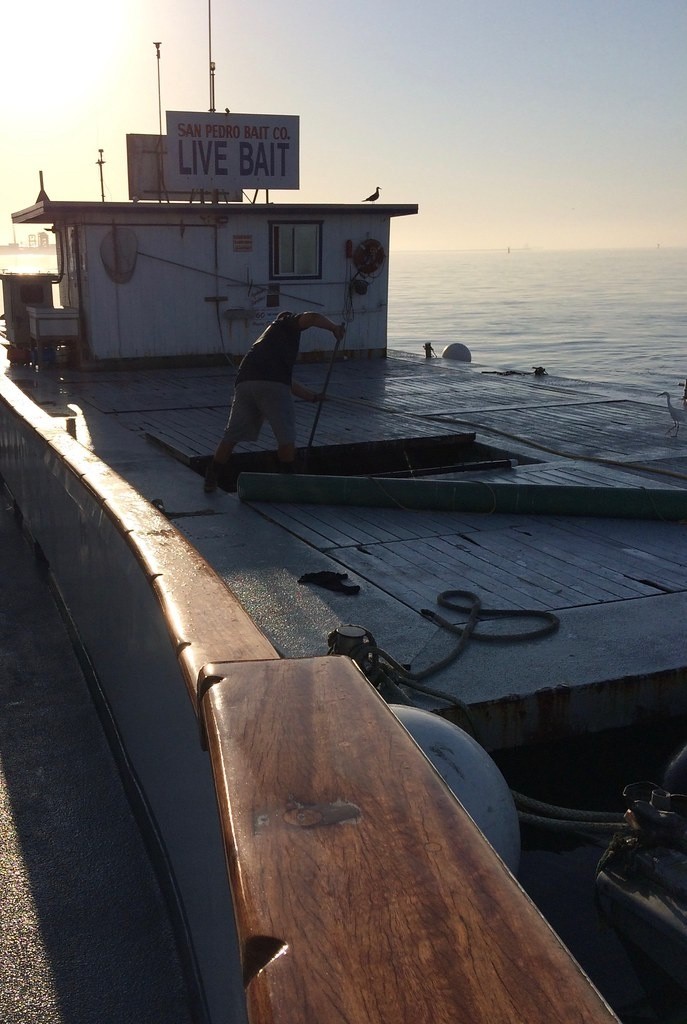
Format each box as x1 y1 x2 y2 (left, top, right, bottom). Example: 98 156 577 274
361 187 382 204
656 391 686 438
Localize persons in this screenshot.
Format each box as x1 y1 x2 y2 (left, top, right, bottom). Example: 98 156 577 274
205 307 344 493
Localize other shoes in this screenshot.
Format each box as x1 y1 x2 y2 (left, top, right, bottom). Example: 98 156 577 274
205 466 216 501
